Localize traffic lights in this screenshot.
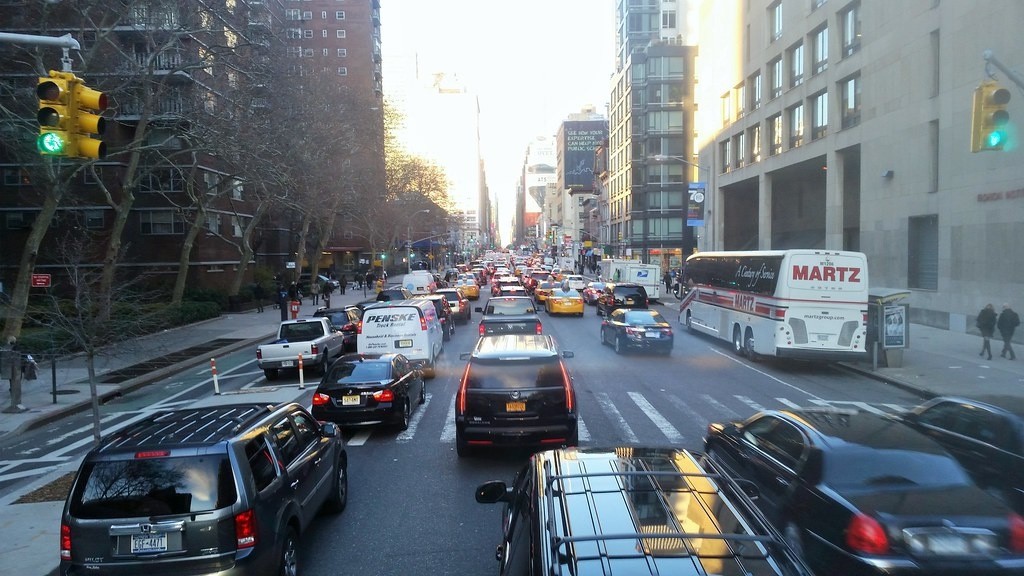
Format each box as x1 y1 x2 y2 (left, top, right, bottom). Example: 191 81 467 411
36 76 107 159
471 235 473 242
382 250 386 259
970 84 1011 153
410 249 413 256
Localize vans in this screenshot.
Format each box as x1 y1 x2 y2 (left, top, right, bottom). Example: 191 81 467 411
357 300 446 378
402 270 434 294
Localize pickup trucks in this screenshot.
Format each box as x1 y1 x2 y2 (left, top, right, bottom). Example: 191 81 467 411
257 316 346 381
474 296 542 337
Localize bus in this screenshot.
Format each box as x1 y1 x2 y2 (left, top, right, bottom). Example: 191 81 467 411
673 249 869 359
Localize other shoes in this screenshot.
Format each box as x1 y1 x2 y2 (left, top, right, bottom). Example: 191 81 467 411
987 355 992 360
1008 356 1015 360
261 311 263 313
1001 353 1006 358
257 311 260 313
979 351 985 356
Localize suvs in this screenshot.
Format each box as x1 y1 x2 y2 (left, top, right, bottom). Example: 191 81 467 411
60 335 814 576
596 282 648 317
313 287 471 352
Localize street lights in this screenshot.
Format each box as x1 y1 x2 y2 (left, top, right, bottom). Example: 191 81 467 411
551 224 603 259
654 155 715 251
407 210 429 273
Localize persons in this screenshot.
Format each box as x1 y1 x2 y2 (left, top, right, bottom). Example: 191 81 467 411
280 268 387 308
977 304 997 360
419 261 430 271
997 303 1019 359
253 281 264 313
579 262 598 273
6 336 27 391
662 268 681 294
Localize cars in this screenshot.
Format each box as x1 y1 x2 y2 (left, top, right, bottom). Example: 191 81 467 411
703 394 1024 576
297 274 340 294
311 352 425 430
435 250 676 355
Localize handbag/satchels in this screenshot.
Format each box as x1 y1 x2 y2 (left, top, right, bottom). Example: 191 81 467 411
322 293 324 299
